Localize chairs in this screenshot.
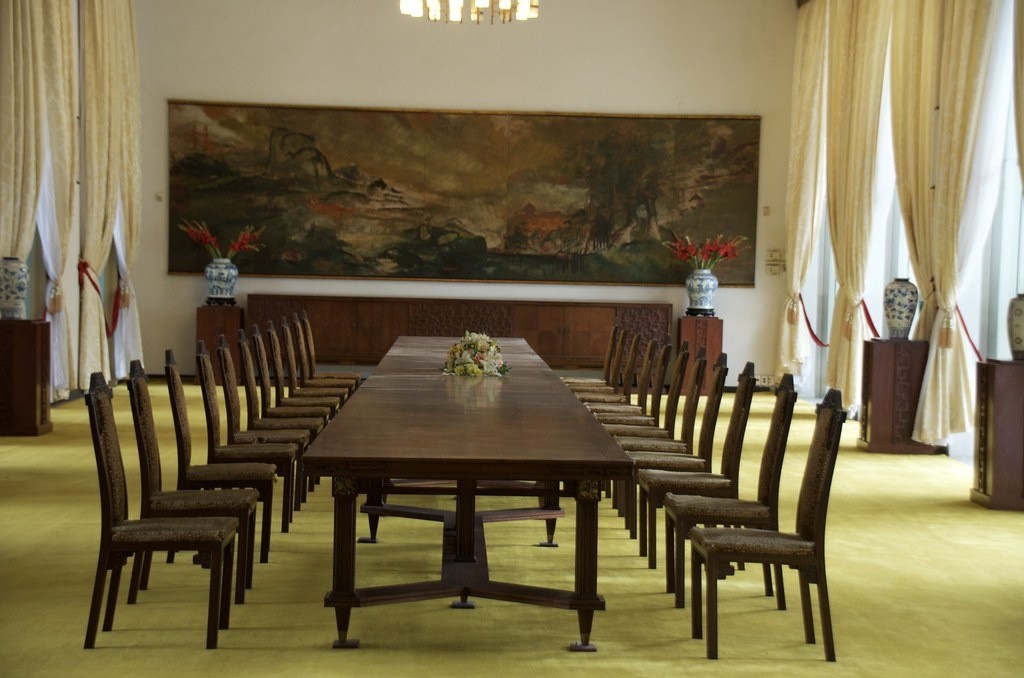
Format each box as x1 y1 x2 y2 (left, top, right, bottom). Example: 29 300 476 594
561 324 847 662
84 308 365 651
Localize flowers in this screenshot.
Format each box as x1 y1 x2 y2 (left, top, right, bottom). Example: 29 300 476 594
439 330 512 377
177 219 268 259
661 230 752 270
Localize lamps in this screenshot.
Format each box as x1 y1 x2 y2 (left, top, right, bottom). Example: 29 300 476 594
398 0 540 22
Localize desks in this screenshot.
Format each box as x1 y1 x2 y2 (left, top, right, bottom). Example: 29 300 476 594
300 330 635 657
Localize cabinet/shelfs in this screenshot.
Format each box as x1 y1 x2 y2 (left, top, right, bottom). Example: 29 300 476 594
968 358 1024 511
676 316 723 396
194 306 244 386
857 338 937 453
0 318 53 437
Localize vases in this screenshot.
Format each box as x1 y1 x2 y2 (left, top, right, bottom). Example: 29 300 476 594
0 257 30 319
882 278 918 338
685 269 719 316
204 259 239 307
1006 293 1024 361
